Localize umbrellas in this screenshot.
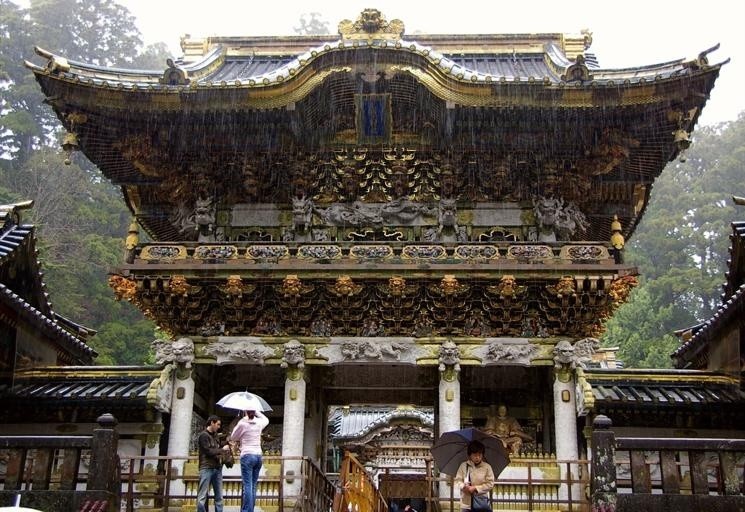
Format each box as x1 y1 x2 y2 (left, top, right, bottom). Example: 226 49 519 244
216 390 274 417
429 427 511 486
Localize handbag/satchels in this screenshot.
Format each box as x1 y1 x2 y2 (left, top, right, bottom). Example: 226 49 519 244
223 448 233 468
471 494 492 512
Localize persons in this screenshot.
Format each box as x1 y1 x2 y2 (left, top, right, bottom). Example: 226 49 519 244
455 440 495 512
403 505 416 512
230 411 270 512
484 405 524 453
196 415 231 512
